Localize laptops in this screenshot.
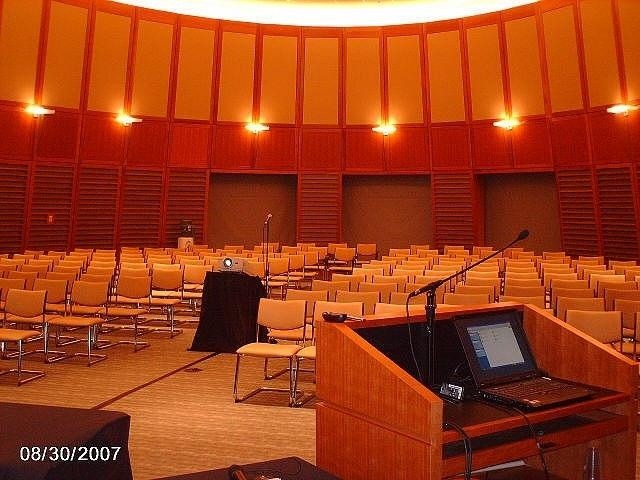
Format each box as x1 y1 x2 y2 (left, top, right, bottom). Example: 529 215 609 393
450 306 596 410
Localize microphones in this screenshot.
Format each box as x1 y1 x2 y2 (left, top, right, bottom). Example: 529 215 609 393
264 213 273 226
407 228 530 293
